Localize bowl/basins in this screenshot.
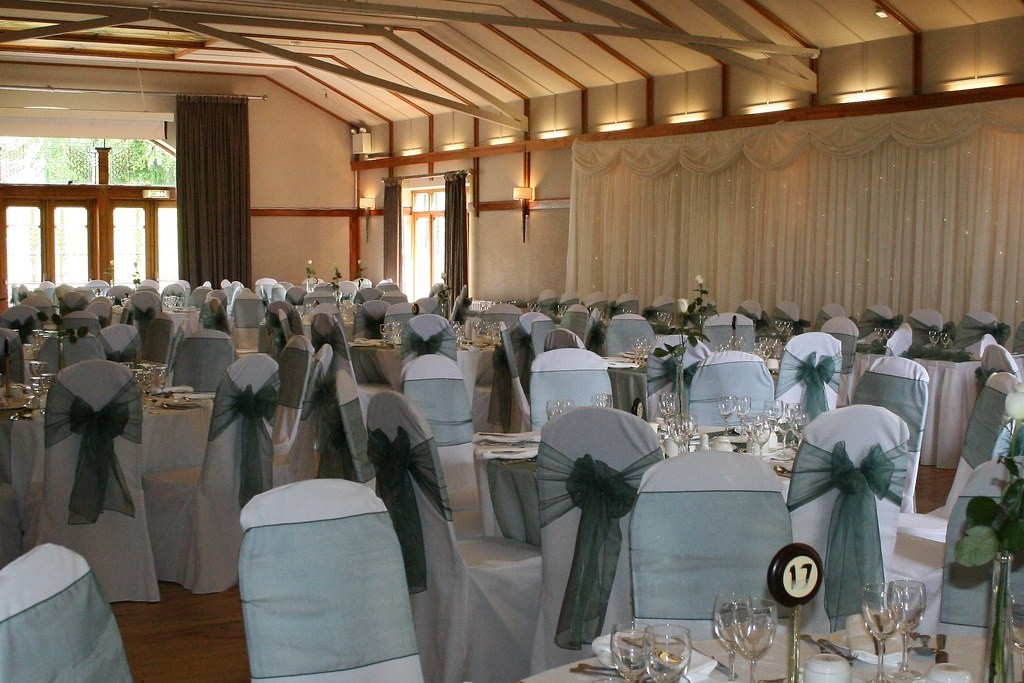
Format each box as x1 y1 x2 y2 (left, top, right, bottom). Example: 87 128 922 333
926 663 974 683
803 654 853 683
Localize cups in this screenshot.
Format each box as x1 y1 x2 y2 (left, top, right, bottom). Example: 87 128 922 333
591 393 613 408
546 400 575 421
609 620 692 683
1009 593 1024 683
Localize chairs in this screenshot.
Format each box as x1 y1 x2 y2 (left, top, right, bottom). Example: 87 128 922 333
0 275 1024 683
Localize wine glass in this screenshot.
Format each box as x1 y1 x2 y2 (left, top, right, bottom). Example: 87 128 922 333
380 321 401 347
294 305 304 321
120 361 169 410
874 327 893 348
29 363 55 414
32 330 50 349
631 337 653 365
473 321 502 346
753 320 794 364
449 321 468 351
659 391 809 461
713 592 777 683
861 580 927 683
163 296 184 311
928 331 951 349
715 336 745 353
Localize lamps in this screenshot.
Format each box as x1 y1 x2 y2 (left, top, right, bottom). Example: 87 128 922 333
875 6 888 19
349 127 373 155
359 197 375 244
513 185 534 244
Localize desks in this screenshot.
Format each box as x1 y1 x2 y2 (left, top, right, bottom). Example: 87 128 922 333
847 344 1024 469
258 302 355 353
295 281 337 294
344 333 531 435
596 311 701 356
457 301 567 325
601 352 779 420
688 323 817 341
469 424 804 550
518 626 1024 683
109 306 203 339
0 382 217 485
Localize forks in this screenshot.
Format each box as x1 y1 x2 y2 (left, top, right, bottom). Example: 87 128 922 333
818 638 858 662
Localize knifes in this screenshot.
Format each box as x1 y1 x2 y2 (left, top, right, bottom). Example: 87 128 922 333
675 637 737 679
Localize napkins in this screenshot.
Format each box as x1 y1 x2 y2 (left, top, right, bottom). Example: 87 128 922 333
471 429 543 444
172 391 217 401
715 430 778 443
474 445 540 460
589 630 719 683
348 337 383 347
843 610 909 668
153 385 195 394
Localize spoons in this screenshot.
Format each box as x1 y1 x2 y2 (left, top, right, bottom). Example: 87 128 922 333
503 454 538 464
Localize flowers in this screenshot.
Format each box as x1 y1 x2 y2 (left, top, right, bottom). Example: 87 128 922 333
692 276 724 318
436 271 454 299
102 260 119 280
132 261 141 285
654 297 711 361
358 277 365 291
956 390 1024 618
52 288 90 343
305 259 317 279
357 259 367 276
331 263 344 291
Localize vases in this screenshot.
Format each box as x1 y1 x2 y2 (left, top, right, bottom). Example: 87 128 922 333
56 336 66 380
333 291 344 321
674 361 687 423
305 276 315 293
440 297 449 321
110 280 114 289
981 551 1024 683
700 315 706 345
134 284 138 292
